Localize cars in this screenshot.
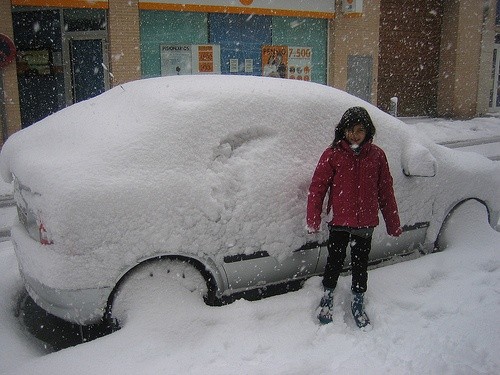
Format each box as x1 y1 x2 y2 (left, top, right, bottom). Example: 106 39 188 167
13 74 500 337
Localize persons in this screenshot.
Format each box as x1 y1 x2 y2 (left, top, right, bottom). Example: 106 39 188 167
263 53 286 79
306 106 403 328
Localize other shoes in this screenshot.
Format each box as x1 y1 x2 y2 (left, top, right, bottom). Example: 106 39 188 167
317 288 334 324
351 293 371 328
